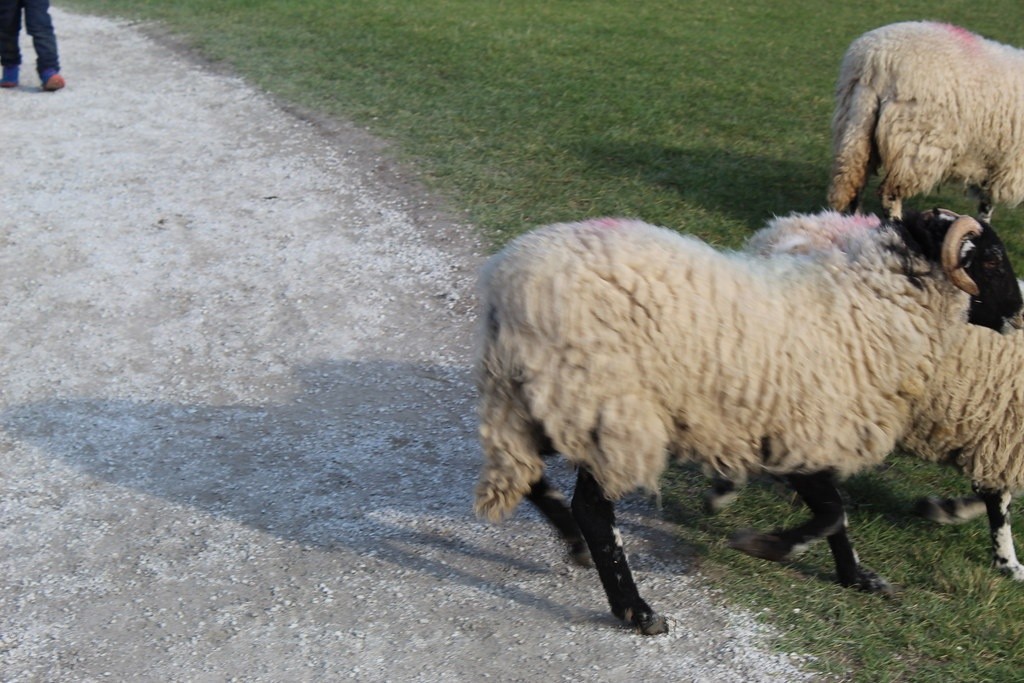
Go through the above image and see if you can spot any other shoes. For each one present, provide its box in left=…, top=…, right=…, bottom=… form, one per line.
left=0, top=64, right=20, bottom=86
left=41, top=67, right=65, bottom=90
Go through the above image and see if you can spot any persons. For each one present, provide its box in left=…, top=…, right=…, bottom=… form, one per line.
left=0, top=0, right=65, bottom=91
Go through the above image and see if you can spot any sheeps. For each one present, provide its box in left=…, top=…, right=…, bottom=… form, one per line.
left=473, top=206, right=1024, bottom=637
left=821, top=13, right=1024, bottom=222
